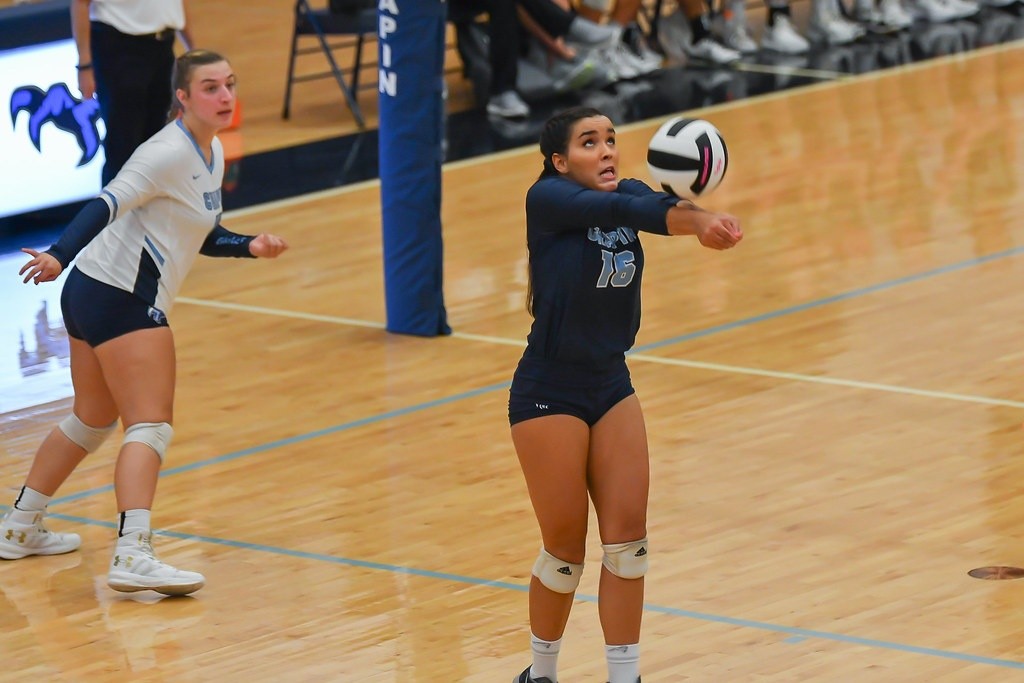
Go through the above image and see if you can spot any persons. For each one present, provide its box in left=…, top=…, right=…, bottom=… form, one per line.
left=507, top=109, right=743, bottom=683
left=68, top=0, right=198, bottom=192
left=444, top=0, right=1024, bottom=118
left=1, top=50, right=290, bottom=597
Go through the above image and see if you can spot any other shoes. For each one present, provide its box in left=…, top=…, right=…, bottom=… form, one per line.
left=810, top=0, right=980, bottom=46
left=543, top=41, right=662, bottom=92
left=487, top=89, right=530, bottom=118
left=717, top=25, right=758, bottom=54
left=682, top=34, right=740, bottom=64
left=567, top=17, right=621, bottom=49
left=760, top=13, right=809, bottom=54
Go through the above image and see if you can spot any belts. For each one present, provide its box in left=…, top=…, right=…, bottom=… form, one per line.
left=91, top=22, right=174, bottom=42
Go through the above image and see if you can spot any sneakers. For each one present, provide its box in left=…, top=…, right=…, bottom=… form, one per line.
left=511, top=663, right=560, bottom=683
left=107, top=530, right=205, bottom=595
left=0, top=506, right=80, bottom=560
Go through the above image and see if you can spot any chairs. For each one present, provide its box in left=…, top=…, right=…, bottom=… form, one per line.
left=281, top=0, right=378, bottom=131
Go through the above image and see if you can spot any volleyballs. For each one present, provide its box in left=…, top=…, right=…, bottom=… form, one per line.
left=648, top=114, right=731, bottom=197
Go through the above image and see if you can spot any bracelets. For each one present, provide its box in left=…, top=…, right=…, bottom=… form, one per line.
left=75, top=61, right=92, bottom=70
